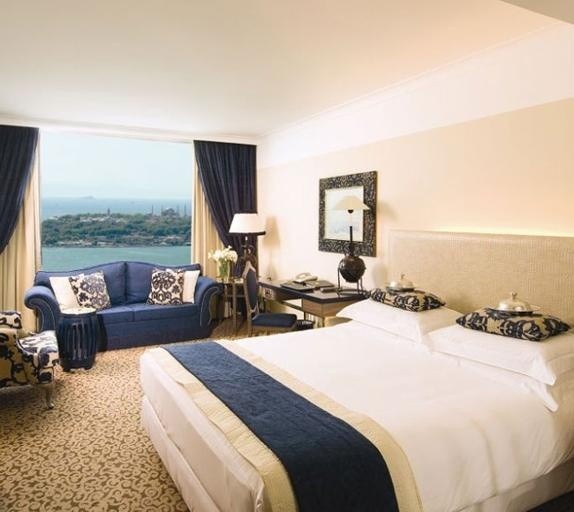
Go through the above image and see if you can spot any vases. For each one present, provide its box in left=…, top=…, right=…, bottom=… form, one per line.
left=216, top=262, right=229, bottom=284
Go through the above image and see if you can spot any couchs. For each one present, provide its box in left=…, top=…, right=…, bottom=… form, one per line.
left=24, top=261, right=224, bottom=350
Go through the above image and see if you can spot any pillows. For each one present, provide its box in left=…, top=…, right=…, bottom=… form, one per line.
left=35, top=260, right=201, bottom=311
left=457, top=306, right=571, bottom=340
left=369, top=288, right=448, bottom=312
left=422, top=320, right=574, bottom=385
left=335, top=297, right=465, bottom=340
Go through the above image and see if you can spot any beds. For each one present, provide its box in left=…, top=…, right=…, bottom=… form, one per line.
left=138, top=233, right=574, bottom=512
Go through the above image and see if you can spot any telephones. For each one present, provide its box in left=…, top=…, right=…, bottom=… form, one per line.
left=294, top=272, right=318, bottom=284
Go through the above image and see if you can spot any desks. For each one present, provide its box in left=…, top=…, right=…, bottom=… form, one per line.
left=257, top=280, right=367, bottom=327
left=214, top=277, right=270, bottom=336
left=56, top=307, right=99, bottom=371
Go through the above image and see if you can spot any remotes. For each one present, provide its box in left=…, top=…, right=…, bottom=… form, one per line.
left=320, top=285, right=342, bottom=293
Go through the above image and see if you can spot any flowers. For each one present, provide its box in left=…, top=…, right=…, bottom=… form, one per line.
left=207, top=245, right=238, bottom=281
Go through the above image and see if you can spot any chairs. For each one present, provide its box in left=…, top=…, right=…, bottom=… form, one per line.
left=242, top=261, right=298, bottom=335
left=0, top=310, right=59, bottom=409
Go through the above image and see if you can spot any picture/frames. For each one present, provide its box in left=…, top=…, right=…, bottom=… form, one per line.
left=318, top=170, right=377, bottom=257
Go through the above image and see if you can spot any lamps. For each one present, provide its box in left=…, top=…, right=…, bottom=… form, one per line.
left=227, top=213, right=266, bottom=284
left=331, top=195, right=371, bottom=297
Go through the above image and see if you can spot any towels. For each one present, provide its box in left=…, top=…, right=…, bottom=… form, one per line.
left=153, top=337, right=423, bottom=512
